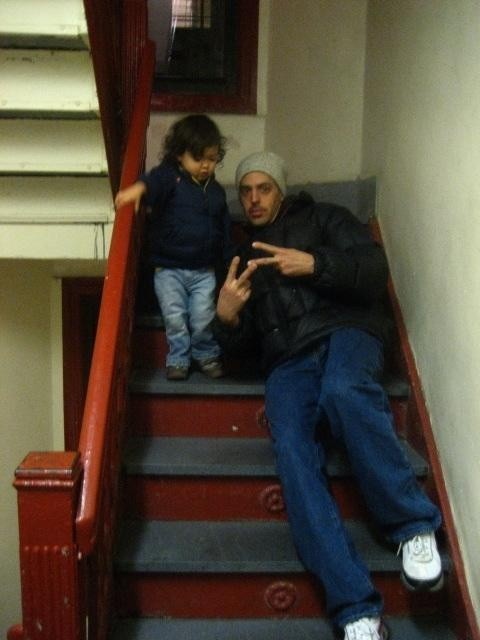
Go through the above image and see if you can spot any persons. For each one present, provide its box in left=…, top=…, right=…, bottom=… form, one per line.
left=216, top=152, right=444, bottom=640
left=115, top=115, right=226, bottom=377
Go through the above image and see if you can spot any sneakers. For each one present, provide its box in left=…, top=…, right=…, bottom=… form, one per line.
left=343, top=616, right=389, bottom=640
left=200, top=358, right=225, bottom=377
left=402, top=530, right=445, bottom=592
left=166, top=366, right=188, bottom=379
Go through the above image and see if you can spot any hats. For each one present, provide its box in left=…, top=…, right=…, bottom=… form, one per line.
left=235, top=152, right=289, bottom=196
